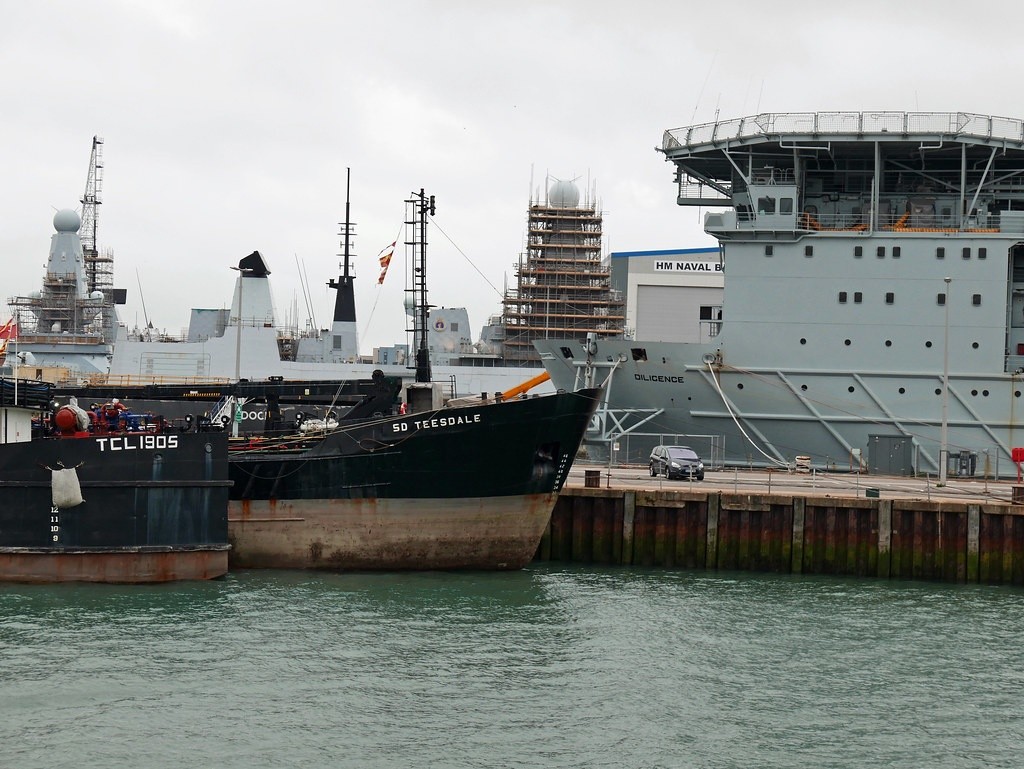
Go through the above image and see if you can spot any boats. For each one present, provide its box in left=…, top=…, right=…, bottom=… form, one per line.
left=0, top=133, right=607, bottom=584
left=530, top=106, right=1024, bottom=481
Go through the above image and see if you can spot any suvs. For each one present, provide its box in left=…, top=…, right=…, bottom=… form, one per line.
left=648, top=444, right=705, bottom=481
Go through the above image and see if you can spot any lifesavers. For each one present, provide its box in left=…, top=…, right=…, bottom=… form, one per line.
left=400, top=402, right=407, bottom=414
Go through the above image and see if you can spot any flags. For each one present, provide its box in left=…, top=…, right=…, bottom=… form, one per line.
left=1, top=310, right=18, bottom=405
left=377, top=240, right=396, bottom=282
